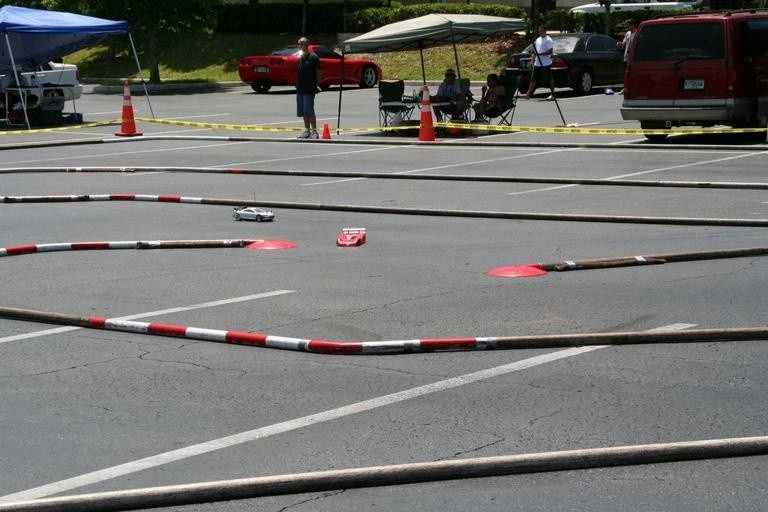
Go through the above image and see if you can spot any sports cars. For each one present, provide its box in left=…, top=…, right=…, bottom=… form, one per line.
left=238, top=44, right=382, bottom=94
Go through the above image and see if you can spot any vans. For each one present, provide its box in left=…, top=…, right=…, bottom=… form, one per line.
left=620, top=9, right=768, bottom=143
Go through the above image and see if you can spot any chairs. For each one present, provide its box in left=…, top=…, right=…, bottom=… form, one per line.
left=372, top=70, right=522, bottom=139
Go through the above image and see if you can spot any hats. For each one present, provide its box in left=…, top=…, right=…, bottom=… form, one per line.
left=445, top=68, right=455, bottom=75
left=11, top=64, right=24, bottom=72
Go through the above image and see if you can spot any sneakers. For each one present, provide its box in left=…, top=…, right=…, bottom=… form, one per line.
left=546, top=94, right=554, bottom=100
left=298, top=131, right=319, bottom=139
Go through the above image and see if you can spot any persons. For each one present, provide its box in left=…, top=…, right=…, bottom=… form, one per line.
left=524, top=25, right=556, bottom=101
left=0, top=63, right=39, bottom=119
left=295, top=37, right=320, bottom=140
left=616, top=19, right=638, bottom=94
left=429, top=68, right=467, bottom=123
left=472, top=74, right=507, bottom=125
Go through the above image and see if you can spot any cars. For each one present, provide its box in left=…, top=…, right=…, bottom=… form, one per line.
left=0, top=56, right=83, bottom=116
left=569, top=0, right=704, bottom=15
left=505, top=33, right=625, bottom=95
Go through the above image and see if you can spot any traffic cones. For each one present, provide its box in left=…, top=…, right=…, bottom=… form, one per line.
left=413, top=85, right=442, bottom=144
left=321, top=123, right=333, bottom=139
left=115, top=80, right=143, bottom=136
